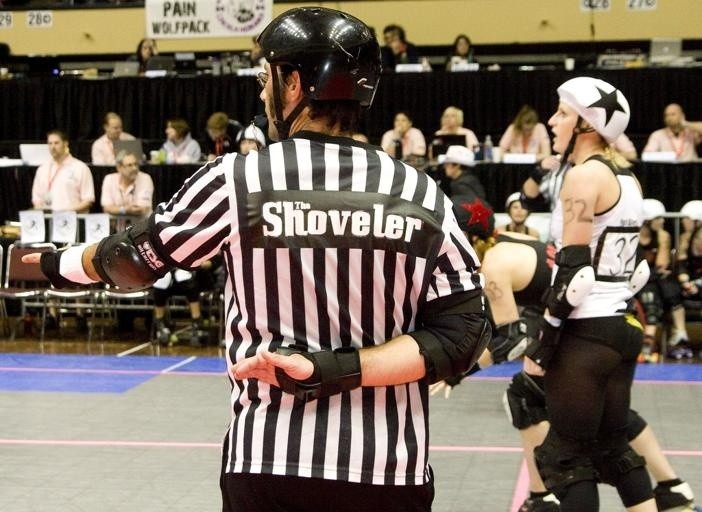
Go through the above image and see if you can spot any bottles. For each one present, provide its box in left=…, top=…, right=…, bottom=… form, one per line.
left=158, top=147, right=167, bottom=164
left=483, top=135, right=493, bottom=163
left=394, top=139, right=402, bottom=161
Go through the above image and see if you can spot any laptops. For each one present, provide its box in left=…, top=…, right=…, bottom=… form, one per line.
left=112, top=61, right=140, bottom=78
left=649, top=37, right=683, bottom=64
left=19, top=143, right=53, bottom=166
left=112, top=138, right=147, bottom=162
left=432, top=133, right=469, bottom=161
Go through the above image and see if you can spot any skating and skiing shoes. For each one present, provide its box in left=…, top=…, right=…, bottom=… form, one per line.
left=155, top=317, right=178, bottom=347
left=638, top=343, right=662, bottom=363
left=668, top=334, right=694, bottom=360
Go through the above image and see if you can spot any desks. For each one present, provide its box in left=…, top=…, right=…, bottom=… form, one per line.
left=1, top=40, right=270, bottom=210
left=359, top=38, right=702, bottom=246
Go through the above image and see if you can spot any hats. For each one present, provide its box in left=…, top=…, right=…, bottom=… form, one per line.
left=438, top=147, right=476, bottom=167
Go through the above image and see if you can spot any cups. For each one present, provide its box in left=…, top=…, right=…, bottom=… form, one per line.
left=150, top=150, right=158, bottom=163
left=565, top=57, right=575, bottom=71
left=491, top=147, right=501, bottom=163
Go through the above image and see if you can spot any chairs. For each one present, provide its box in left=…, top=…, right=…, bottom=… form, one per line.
left=655, top=250, right=702, bottom=346
left=0, top=210, right=226, bottom=347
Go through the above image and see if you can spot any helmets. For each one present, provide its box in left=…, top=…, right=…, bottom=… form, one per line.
left=642, top=198, right=667, bottom=224
left=557, top=76, right=631, bottom=145
left=679, top=199, right=702, bottom=221
left=236, top=126, right=266, bottom=149
left=256, top=8, right=382, bottom=111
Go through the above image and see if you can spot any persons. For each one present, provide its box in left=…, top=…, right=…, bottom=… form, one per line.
left=450, top=194, right=702, bottom=511
left=21, top=6, right=498, bottom=512
left=523, top=76, right=658, bottom=511
